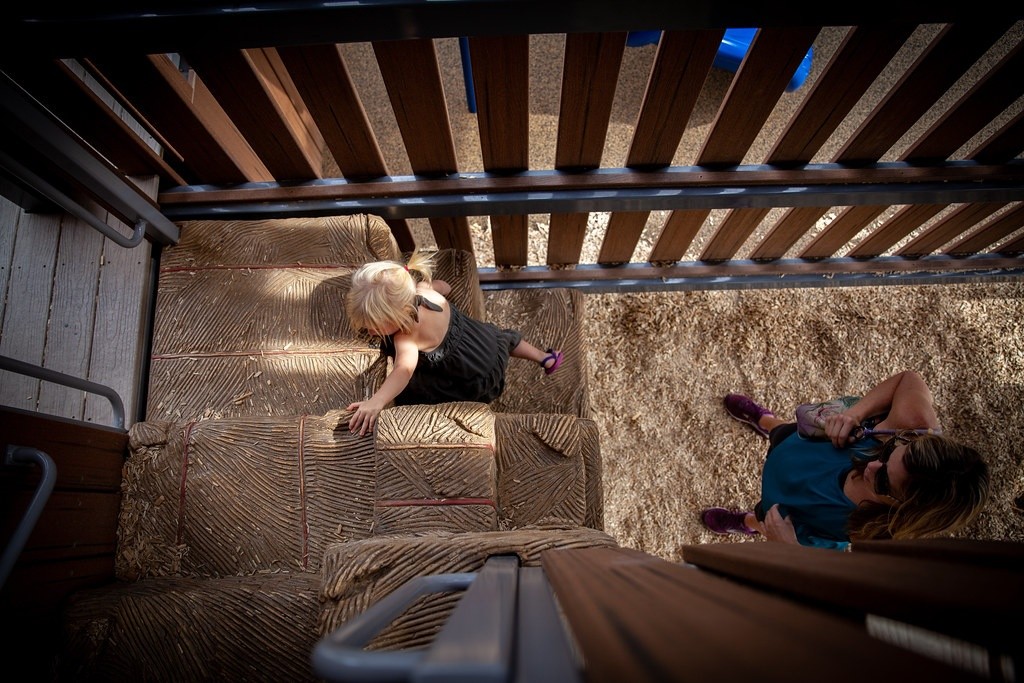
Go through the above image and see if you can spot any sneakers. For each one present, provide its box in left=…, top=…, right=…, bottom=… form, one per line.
left=724, top=393, right=775, bottom=439
left=701, top=506, right=761, bottom=538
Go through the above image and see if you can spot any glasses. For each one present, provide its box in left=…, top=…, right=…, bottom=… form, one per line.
left=873, top=435, right=911, bottom=504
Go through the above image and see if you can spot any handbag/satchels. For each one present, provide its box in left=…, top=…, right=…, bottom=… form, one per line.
left=796, top=395, right=943, bottom=445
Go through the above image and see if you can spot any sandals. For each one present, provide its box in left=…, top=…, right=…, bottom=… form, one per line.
left=540, top=349, right=564, bottom=375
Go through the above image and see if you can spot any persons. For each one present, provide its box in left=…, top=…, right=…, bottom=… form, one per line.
left=699, top=372, right=990, bottom=550
left=346, top=259, right=565, bottom=436
